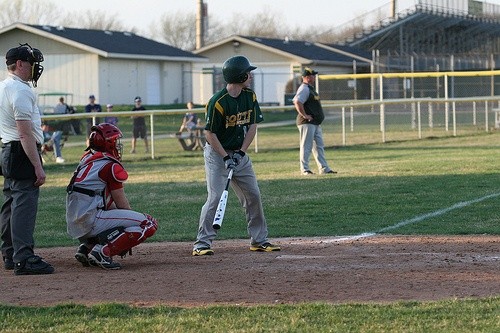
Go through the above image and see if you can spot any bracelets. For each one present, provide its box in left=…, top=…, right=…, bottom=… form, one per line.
left=235, top=149, right=245, bottom=157
left=223, top=155, right=231, bottom=162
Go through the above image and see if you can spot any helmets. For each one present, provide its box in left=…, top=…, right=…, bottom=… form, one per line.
left=222, top=57, right=258, bottom=84
left=89, top=123, right=123, bottom=151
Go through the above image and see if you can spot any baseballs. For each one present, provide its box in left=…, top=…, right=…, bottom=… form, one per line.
left=212, top=170, right=234, bottom=229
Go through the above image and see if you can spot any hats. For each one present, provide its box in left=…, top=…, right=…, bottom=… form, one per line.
left=106, top=104, right=113, bottom=108
left=6, top=48, right=35, bottom=65
left=88, top=95, right=95, bottom=100
left=302, top=68, right=319, bottom=77
left=134, top=96, right=142, bottom=102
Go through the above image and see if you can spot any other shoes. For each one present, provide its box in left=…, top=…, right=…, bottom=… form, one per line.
left=130, top=147, right=149, bottom=154
left=327, top=170, right=337, bottom=174
left=302, top=171, right=316, bottom=175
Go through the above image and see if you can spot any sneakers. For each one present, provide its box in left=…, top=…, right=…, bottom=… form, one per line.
left=192, top=248, right=215, bottom=256
left=14, top=254, right=54, bottom=275
left=56, top=156, right=66, bottom=163
left=75, top=243, right=92, bottom=266
left=88, top=244, right=121, bottom=270
left=4, top=258, right=15, bottom=270
left=249, top=242, right=281, bottom=252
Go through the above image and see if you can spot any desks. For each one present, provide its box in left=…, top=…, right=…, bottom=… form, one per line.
left=175, top=124, right=208, bottom=152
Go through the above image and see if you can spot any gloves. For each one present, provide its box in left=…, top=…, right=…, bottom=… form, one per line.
left=223, top=150, right=246, bottom=169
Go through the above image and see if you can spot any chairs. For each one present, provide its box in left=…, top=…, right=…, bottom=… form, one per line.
left=40, top=135, right=69, bottom=164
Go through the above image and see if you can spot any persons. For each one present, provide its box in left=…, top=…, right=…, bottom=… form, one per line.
left=192, top=56, right=281, bottom=256
left=103, top=104, right=118, bottom=127
left=85, top=95, right=102, bottom=148
left=39, top=97, right=83, bottom=164
left=292, top=68, right=338, bottom=174
left=178, top=102, right=198, bottom=143
left=129, top=97, right=150, bottom=153
left=0, top=42, right=54, bottom=275
left=65, top=123, right=158, bottom=271
